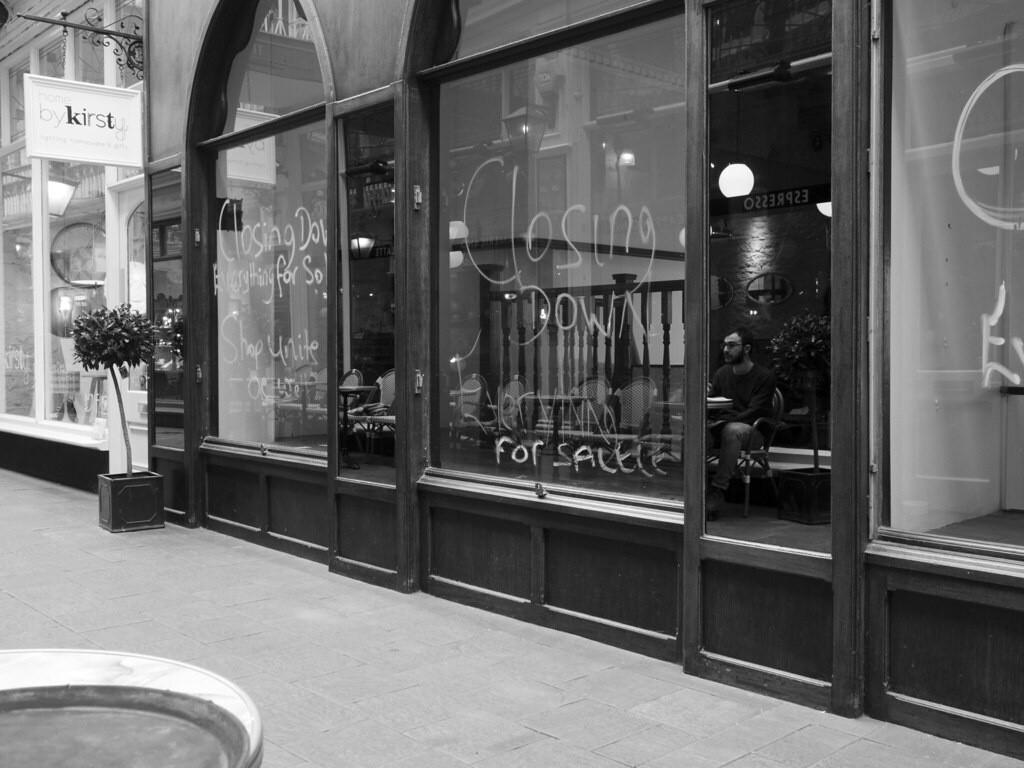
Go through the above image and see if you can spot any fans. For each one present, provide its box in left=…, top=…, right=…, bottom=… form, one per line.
left=50, top=223, right=107, bottom=290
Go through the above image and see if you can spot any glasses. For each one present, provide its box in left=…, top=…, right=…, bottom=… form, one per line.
left=719, top=341, right=744, bottom=349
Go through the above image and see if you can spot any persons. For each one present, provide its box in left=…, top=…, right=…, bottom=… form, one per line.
left=700, top=327, right=775, bottom=513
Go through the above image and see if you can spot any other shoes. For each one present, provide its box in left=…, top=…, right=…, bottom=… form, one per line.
left=706, top=491, right=721, bottom=522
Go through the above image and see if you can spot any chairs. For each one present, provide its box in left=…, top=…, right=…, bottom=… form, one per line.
left=566, top=375, right=664, bottom=477
left=258, top=360, right=396, bottom=473
left=453, top=374, right=528, bottom=465
left=707, top=389, right=782, bottom=518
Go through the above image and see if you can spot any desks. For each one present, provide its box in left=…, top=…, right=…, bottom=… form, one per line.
left=451, top=390, right=479, bottom=447
left=607, top=395, right=735, bottom=487
left=282, top=381, right=327, bottom=442
left=339, top=385, right=375, bottom=470
left=523, top=395, right=594, bottom=466
left=81, top=374, right=109, bottom=425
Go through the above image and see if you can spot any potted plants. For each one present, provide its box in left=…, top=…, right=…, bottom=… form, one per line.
left=772, top=313, right=831, bottom=526
left=72, top=303, right=167, bottom=533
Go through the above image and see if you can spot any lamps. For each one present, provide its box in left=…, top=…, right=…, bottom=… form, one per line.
left=775, top=64, right=794, bottom=80
left=718, top=94, right=755, bottom=199
